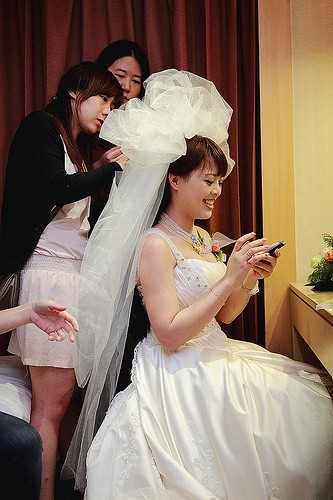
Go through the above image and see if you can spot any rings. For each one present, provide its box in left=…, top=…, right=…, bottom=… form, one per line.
left=245, top=250, right=252, bottom=258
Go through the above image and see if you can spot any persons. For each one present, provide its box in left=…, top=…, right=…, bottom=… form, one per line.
left=0, top=300, right=79, bottom=500
left=91, top=39, right=150, bottom=169
left=0, top=61, right=132, bottom=500
left=84, top=135, right=333, bottom=500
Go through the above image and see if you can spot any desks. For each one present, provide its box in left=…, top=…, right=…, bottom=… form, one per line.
left=288, top=282, right=333, bottom=379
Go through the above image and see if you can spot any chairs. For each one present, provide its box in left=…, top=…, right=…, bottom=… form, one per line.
left=0, top=411, right=45, bottom=500
left=0, top=354, right=34, bottom=423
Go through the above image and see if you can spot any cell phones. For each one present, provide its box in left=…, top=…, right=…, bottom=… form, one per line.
left=258, top=243, right=286, bottom=264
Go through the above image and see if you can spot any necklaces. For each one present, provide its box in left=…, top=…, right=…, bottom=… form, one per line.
left=158, top=212, right=207, bottom=258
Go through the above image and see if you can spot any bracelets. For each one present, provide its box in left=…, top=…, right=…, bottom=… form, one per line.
left=240, top=283, right=252, bottom=292
left=210, top=288, right=225, bottom=305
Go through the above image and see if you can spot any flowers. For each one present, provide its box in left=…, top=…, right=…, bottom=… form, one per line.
left=197, top=227, right=224, bottom=263
left=303, top=232, right=333, bottom=293
